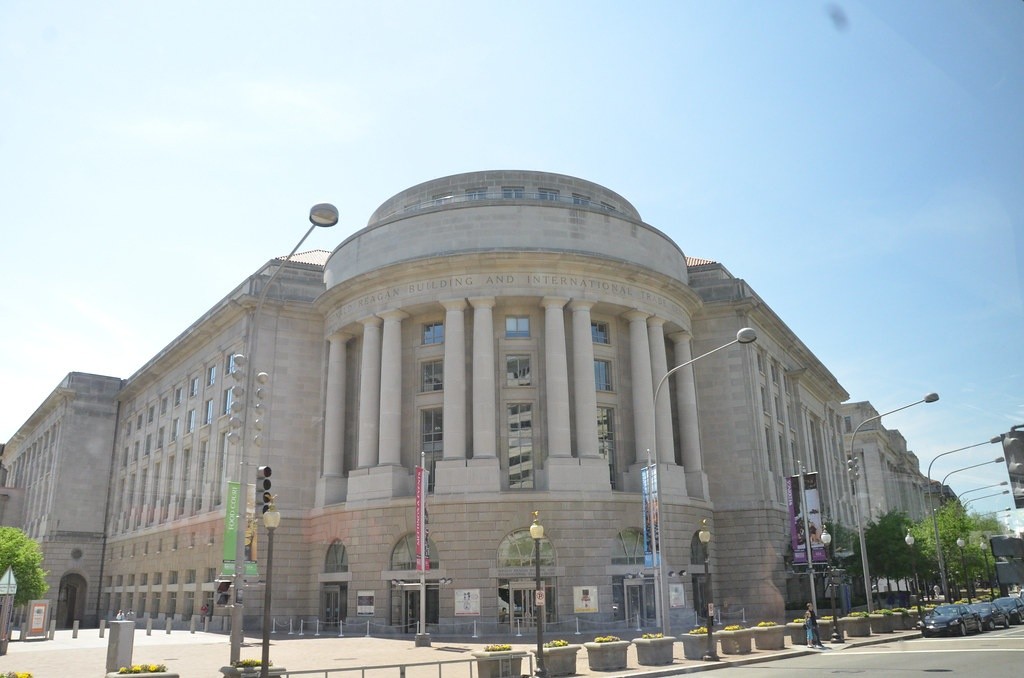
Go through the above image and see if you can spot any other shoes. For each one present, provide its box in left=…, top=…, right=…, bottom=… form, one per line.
left=816, top=644, right=822, bottom=648
left=808, top=644, right=813, bottom=647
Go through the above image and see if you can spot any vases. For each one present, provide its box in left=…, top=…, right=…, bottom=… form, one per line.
left=104, top=670, right=179, bottom=678
left=632, top=635, right=677, bottom=665
left=471, top=650, right=527, bottom=678
left=584, top=640, right=630, bottom=671
left=753, top=624, right=787, bottom=650
left=787, top=610, right=934, bottom=644
left=530, top=644, right=580, bottom=677
left=221, top=666, right=286, bottom=678
left=682, top=631, right=721, bottom=659
left=717, top=627, right=758, bottom=654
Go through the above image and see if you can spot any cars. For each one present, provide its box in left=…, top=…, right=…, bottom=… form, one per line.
left=992, top=589, right=1024, bottom=625
left=919, top=603, right=984, bottom=637
left=967, top=601, right=1011, bottom=631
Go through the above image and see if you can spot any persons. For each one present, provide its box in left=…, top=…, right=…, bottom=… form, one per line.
left=805, top=603, right=822, bottom=648
left=809, top=522, right=816, bottom=542
left=201, top=603, right=212, bottom=629
left=116, top=610, right=125, bottom=621
left=126, top=609, right=136, bottom=620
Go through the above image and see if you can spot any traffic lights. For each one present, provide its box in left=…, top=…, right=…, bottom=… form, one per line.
left=214, top=579, right=233, bottom=605
left=254, top=464, right=272, bottom=518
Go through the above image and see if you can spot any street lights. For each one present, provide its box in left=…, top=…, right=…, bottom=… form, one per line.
left=850, top=394, right=941, bottom=614
left=904, top=531, right=923, bottom=620
left=820, top=525, right=844, bottom=642
left=260, top=494, right=282, bottom=678
left=529, top=510, right=552, bottom=678
left=956, top=537, right=972, bottom=604
left=228, top=203, right=342, bottom=669
left=651, top=327, right=758, bottom=641
left=980, top=542, right=996, bottom=598
left=939, top=456, right=1006, bottom=508
left=697, top=518, right=720, bottom=662
left=928, top=436, right=1003, bottom=603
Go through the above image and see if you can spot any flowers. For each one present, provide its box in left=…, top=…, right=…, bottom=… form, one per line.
left=724, top=623, right=744, bottom=631
left=793, top=594, right=994, bottom=623
left=484, top=643, right=512, bottom=652
left=757, top=621, right=779, bottom=627
left=689, top=626, right=712, bottom=634
left=0, top=671, right=34, bottom=678
left=595, top=635, right=621, bottom=643
left=642, top=632, right=665, bottom=639
left=118, top=661, right=167, bottom=675
left=543, top=638, right=568, bottom=647
left=231, top=659, right=271, bottom=667
